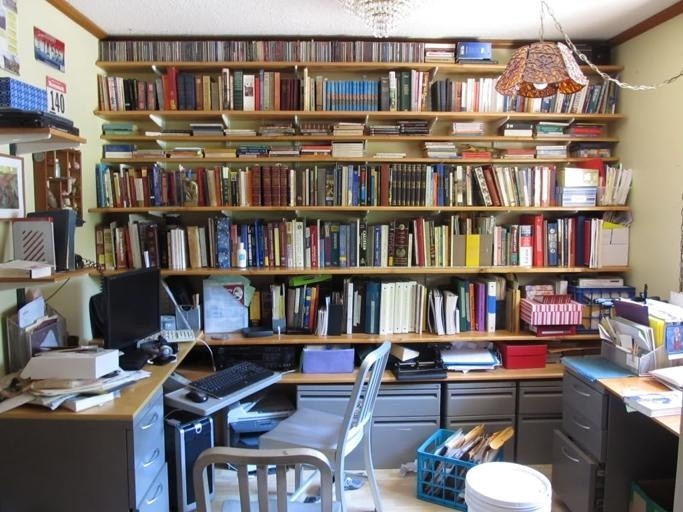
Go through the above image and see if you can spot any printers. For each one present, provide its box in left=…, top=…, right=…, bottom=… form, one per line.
left=224, top=393, right=296, bottom=474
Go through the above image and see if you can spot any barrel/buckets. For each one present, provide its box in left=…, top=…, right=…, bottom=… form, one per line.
left=464, top=461, right=554, bottom=512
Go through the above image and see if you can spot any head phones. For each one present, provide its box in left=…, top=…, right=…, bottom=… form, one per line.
left=140, top=340, right=178, bottom=358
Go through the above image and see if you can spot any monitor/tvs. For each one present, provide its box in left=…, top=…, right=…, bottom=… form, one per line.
left=103, top=267, right=161, bottom=370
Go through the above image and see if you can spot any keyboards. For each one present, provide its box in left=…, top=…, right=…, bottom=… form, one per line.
left=187, top=360, right=274, bottom=400
left=137, top=329, right=195, bottom=348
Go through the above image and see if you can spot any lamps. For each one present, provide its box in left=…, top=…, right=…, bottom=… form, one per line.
left=494, top=3, right=588, bottom=100
left=335, top=0, right=415, bottom=38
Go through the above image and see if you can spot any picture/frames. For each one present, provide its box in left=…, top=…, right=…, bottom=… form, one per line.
left=0, top=153, right=27, bottom=222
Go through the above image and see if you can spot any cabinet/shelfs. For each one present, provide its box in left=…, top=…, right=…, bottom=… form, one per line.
left=32, top=148, right=83, bottom=227
left=1, top=126, right=97, bottom=293
left=88, top=38, right=636, bottom=385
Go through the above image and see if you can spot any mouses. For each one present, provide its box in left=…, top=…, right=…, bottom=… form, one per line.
left=187, top=390, right=208, bottom=403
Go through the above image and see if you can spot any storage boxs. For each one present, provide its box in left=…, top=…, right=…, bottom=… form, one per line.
left=627, top=477, right=676, bottom=512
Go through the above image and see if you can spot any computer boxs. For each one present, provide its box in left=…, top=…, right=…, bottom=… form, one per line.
left=165, top=410, right=215, bottom=512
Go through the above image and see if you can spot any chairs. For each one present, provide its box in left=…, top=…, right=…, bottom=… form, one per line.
left=193, top=445, right=334, bottom=512
left=258, top=339, right=391, bottom=511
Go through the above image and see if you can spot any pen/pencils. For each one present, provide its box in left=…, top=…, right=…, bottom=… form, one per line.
left=192, top=292, right=199, bottom=308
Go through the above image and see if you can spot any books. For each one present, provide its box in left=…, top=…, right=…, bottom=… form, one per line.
left=95, top=160, right=633, bottom=208
left=98, top=40, right=493, bottom=63
left=0, top=346, right=151, bottom=413
left=98, top=69, right=618, bottom=114
left=96, top=214, right=629, bottom=270
left=597, top=291, right=683, bottom=417
left=103, top=122, right=612, bottom=159
left=204, top=274, right=630, bottom=335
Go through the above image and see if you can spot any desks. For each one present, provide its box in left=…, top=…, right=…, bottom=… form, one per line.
left=1, top=328, right=204, bottom=512
left=551, top=355, right=682, bottom=512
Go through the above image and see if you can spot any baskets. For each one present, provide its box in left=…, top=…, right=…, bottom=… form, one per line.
left=417, top=430, right=504, bottom=511
